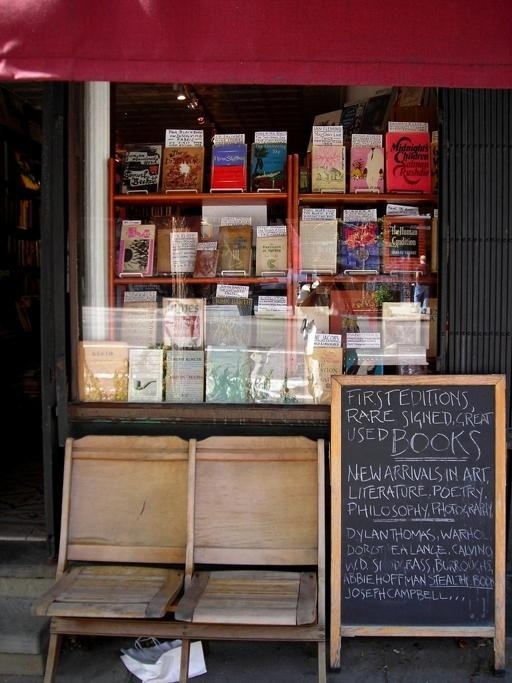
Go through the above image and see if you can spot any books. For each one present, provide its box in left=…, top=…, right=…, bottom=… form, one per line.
left=75, top=87, right=439, bottom=408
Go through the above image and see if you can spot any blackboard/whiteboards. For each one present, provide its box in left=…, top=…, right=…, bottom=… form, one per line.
left=340, top=386, right=496, bottom=628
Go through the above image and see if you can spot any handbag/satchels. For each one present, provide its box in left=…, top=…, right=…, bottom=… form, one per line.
left=120, top=639, right=208, bottom=683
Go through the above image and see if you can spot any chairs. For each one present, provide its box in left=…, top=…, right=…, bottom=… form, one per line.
left=30, top=434, right=327, bottom=682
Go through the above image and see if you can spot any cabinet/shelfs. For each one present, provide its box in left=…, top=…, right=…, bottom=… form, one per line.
left=107, top=154, right=439, bottom=377
